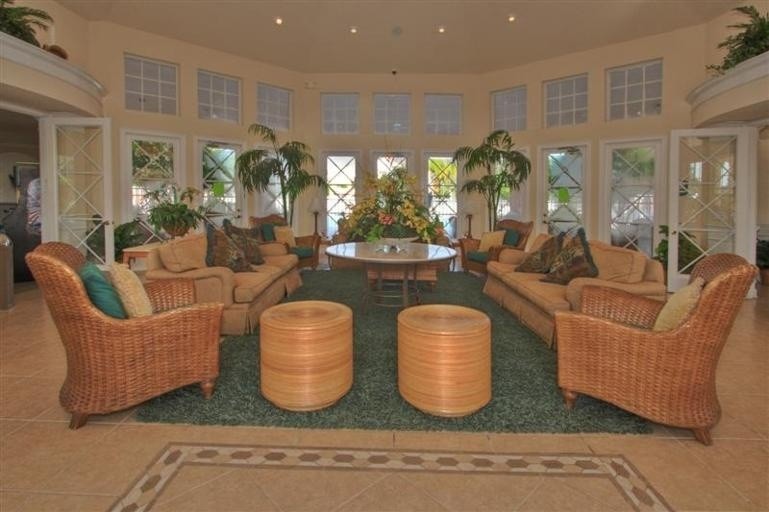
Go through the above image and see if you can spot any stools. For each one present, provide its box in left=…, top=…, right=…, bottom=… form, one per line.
left=397, top=304, right=493, bottom=418
left=258, top=301, right=354, bottom=412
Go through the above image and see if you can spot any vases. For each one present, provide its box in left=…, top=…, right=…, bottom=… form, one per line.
left=381, top=236, right=420, bottom=243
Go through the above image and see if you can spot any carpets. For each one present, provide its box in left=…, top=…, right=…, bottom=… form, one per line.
left=134, top=270, right=656, bottom=436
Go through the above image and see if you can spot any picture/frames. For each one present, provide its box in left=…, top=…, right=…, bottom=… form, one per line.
left=15, top=161, right=40, bottom=189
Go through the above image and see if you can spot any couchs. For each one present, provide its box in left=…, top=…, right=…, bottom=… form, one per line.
left=124, top=229, right=302, bottom=336
left=483, top=232, right=668, bottom=353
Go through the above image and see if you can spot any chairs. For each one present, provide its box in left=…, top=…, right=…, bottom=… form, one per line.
left=554, top=253, right=760, bottom=445
left=249, top=215, right=321, bottom=270
left=25, top=242, right=225, bottom=430
left=458, top=218, right=534, bottom=276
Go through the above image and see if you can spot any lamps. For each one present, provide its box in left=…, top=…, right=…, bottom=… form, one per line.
left=308, top=198, right=322, bottom=235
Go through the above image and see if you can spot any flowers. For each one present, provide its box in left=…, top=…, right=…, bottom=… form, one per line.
left=335, top=165, right=446, bottom=245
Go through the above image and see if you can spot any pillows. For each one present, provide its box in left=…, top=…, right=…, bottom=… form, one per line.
left=206, top=219, right=298, bottom=272
left=78, top=258, right=126, bottom=319
left=653, top=275, right=706, bottom=331
left=478, top=227, right=598, bottom=285
left=109, top=261, right=152, bottom=319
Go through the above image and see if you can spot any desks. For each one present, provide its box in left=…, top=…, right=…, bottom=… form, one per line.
left=122, top=241, right=166, bottom=269
left=367, top=264, right=438, bottom=294
left=325, top=241, right=457, bottom=309
